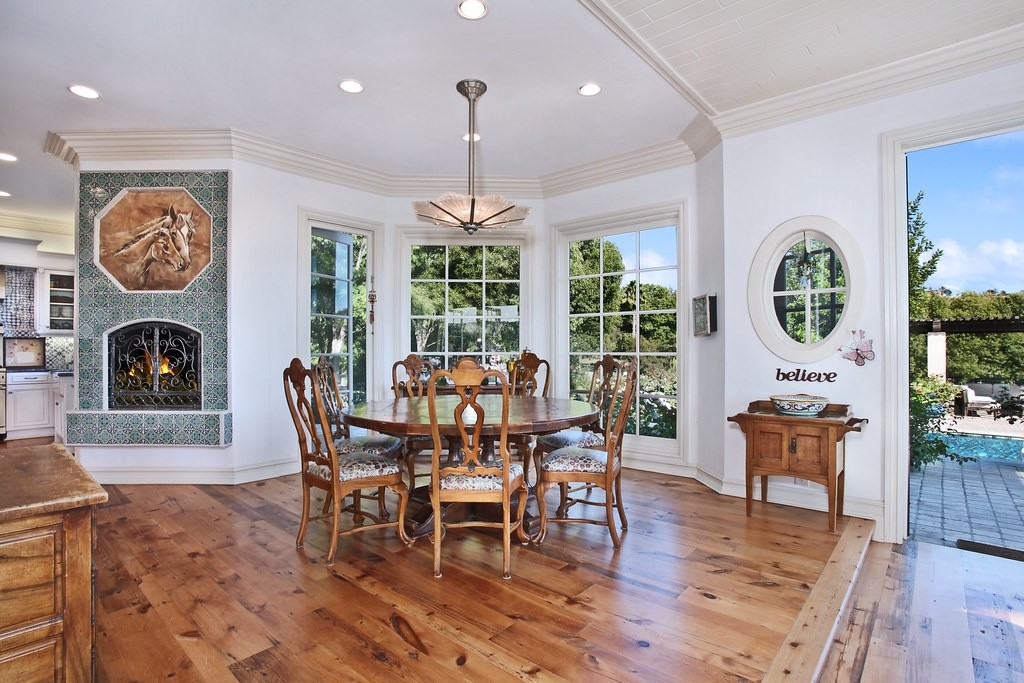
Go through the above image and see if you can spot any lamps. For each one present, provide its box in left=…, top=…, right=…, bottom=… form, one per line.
left=411, top=79, right=531, bottom=235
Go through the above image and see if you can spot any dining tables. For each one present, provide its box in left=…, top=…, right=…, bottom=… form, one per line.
left=390, top=382, right=537, bottom=398
left=339, top=392, right=602, bottom=543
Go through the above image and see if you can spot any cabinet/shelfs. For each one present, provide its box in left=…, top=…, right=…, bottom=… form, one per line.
left=44, top=269, right=74, bottom=334
left=726, top=400, right=868, bottom=533
left=1, top=443, right=109, bottom=683
left=54, top=377, right=74, bottom=443
left=6, top=371, right=55, bottom=440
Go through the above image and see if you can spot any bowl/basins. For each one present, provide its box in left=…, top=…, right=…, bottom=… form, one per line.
left=769, top=394, right=829, bottom=416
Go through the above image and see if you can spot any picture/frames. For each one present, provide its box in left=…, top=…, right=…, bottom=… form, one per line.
left=92, top=186, right=214, bottom=294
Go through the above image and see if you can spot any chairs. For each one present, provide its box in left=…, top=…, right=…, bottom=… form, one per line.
left=312, top=355, right=407, bottom=523
left=426, top=356, right=530, bottom=580
left=283, top=357, right=415, bottom=567
left=532, top=354, right=623, bottom=495
left=531, top=363, right=637, bottom=551
left=495, top=351, right=551, bottom=489
left=392, top=354, right=450, bottom=499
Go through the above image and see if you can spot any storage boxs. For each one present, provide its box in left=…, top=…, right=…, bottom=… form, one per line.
left=768, top=393, right=830, bottom=418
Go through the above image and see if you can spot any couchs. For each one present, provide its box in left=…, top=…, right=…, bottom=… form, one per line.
left=954, top=383, right=1024, bottom=419
